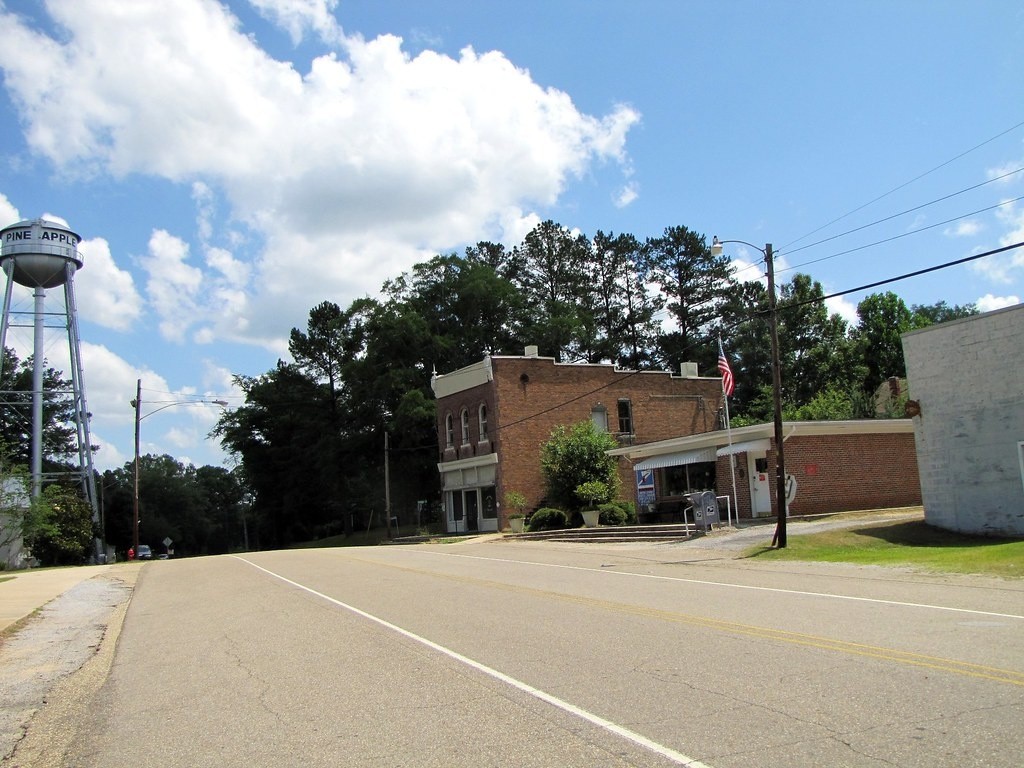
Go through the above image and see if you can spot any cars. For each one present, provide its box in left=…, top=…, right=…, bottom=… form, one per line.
left=136, top=545, right=153, bottom=559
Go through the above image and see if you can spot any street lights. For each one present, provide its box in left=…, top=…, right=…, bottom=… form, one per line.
left=130, top=399, right=228, bottom=560
left=710, top=236, right=788, bottom=547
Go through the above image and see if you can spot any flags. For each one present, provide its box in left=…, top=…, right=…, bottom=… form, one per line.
left=718, top=336, right=735, bottom=397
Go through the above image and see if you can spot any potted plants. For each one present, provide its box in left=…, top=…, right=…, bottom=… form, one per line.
left=648, top=501, right=656, bottom=512
left=573, top=481, right=608, bottom=528
left=504, top=490, right=527, bottom=533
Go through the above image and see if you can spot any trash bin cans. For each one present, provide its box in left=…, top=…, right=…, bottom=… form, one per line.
left=690, top=491, right=722, bottom=535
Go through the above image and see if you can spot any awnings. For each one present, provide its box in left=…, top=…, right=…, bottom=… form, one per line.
left=632, top=446, right=718, bottom=471
left=716, top=438, right=772, bottom=457
